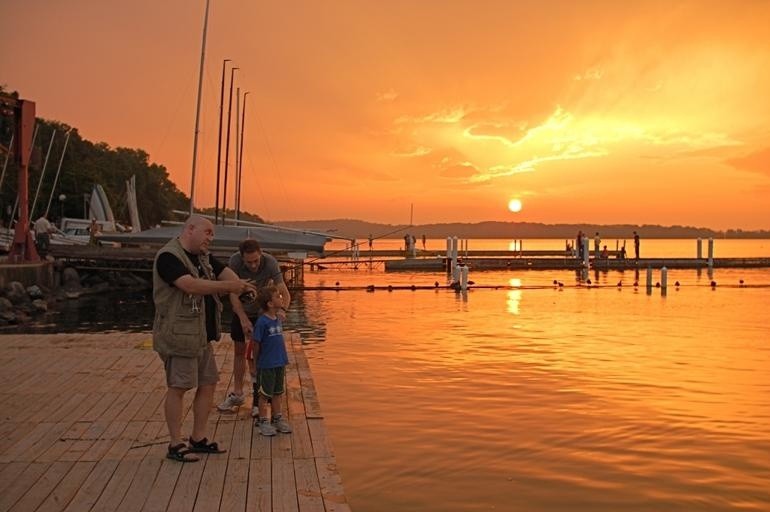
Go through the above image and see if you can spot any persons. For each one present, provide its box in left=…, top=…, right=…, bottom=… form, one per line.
left=617, top=247, right=627, bottom=259
left=403, top=233, right=410, bottom=250
left=33, top=211, right=53, bottom=259
left=150, top=216, right=257, bottom=463
left=632, top=230, right=640, bottom=259
left=368, top=233, right=374, bottom=250
left=576, top=230, right=583, bottom=257
left=601, top=245, right=608, bottom=259
left=594, top=232, right=601, bottom=259
left=248, top=285, right=293, bottom=437
left=581, top=231, right=589, bottom=260
left=86, top=217, right=100, bottom=246
left=214, top=237, right=291, bottom=419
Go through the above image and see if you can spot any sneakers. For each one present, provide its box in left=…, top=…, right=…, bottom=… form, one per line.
left=272, top=417, right=293, bottom=433
left=258, top=421, right=277, bottom=436
left=251, top=406, right=259, bottom=417
left=216, top=393, right=246, bottom=411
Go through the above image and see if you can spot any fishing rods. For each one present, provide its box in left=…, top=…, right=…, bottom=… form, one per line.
left=233, top=222, right=414, bottom=303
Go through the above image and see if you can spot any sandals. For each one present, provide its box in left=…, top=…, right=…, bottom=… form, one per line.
left=188, top=436, right=227, bottom=454
left=166, top=443, right=200, bottom=462
